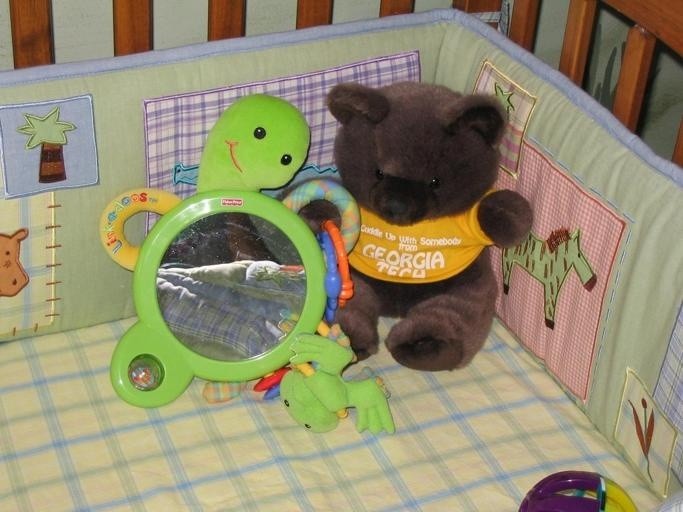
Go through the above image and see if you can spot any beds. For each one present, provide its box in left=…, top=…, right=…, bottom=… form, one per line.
left=0, top=0, right=683, bottom=512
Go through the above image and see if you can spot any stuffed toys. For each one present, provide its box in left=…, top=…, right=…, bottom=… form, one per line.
left=274, top=81, right=531, bottom=370
left=280, top=322, right=395, bottom=435
left=197, top=94, right=310, bottom=193
